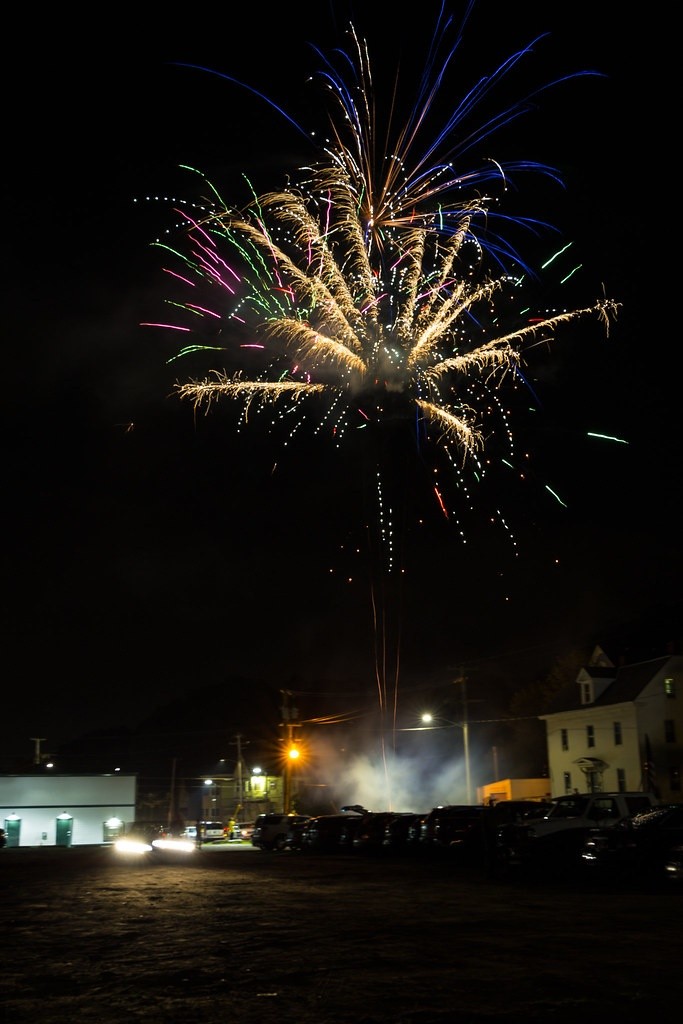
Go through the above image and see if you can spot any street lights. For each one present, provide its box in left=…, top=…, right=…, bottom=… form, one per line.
left=220, top=758, right=242, bottom=806
left=419, top=711, right=474, bottom=806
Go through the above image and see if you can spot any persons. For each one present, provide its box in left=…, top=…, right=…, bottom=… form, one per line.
left=228, top=818, right=236, bottom=840
left=195, top=819, right=203, bottom=849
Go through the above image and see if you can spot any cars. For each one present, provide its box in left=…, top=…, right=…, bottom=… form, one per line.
left=124, top=820, right=173, bottom=865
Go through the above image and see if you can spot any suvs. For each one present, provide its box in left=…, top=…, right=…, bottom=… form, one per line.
left=196, top=820, right=226, bottom=842
left=228, top=791, right=683, bottom=895
